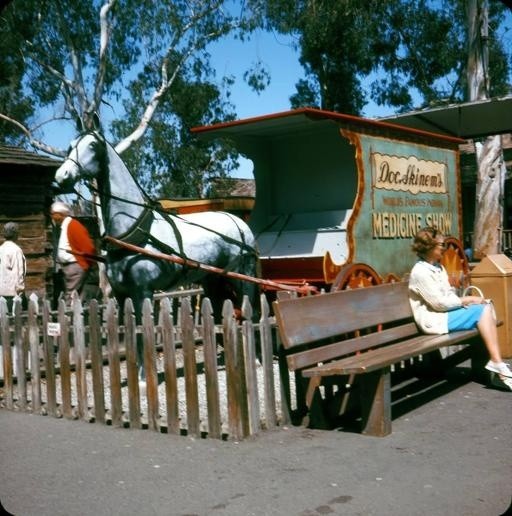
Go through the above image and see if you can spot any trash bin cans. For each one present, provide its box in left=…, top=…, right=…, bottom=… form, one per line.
left=467, top=253, right=512, bottom=358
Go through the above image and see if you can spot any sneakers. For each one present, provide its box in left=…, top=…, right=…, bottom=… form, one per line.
left=485, top=360, right=512, bottom=390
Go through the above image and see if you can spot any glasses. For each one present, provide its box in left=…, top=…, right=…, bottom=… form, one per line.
left=439, top=242, right=445, bottom=247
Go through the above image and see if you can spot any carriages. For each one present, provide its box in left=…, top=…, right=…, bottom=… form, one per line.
left=52, top=106, right=468, bottom=396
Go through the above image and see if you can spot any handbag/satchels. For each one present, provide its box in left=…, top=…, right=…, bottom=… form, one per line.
left=463, top=286, right=496, bottom=321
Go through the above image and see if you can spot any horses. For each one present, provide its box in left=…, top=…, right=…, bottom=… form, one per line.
left=54, top=110, right=263, bottom=387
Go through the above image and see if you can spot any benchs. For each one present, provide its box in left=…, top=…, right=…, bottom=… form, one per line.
left=272, top=279, right=504, bottom=437
left=247, top=208, right=355, bottom=284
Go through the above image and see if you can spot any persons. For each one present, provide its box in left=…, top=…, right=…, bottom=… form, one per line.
left=408, top=227, right=511, bottom=391
left=1, top=222, right=28, bottom=342
left=40, top=201, right=95, bottom=365
